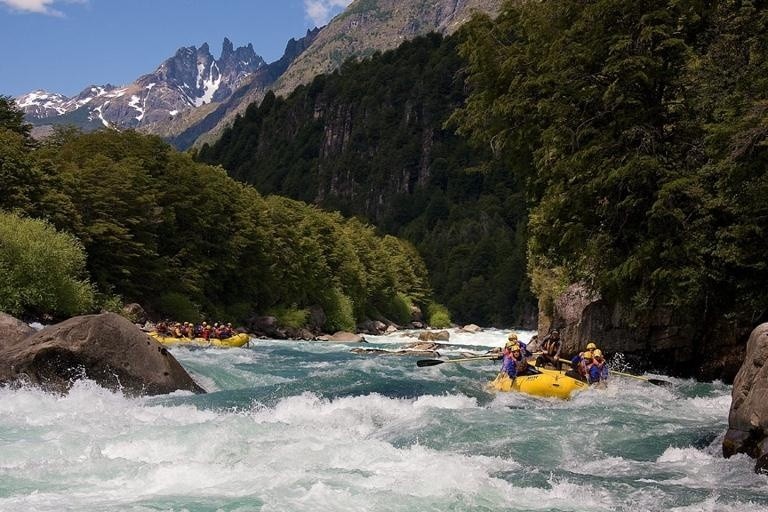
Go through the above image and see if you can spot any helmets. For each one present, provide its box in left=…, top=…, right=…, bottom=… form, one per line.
left=175, top=321, right=232, bottom=330
left=511, top=345, right=520, bottom=352
left=508, top=333, right=519, bottom=341
left=586, top=342, right=597, bottom=349
left=505, top=341, right=514, bottom=347
left=583, top=351, right=592, bottom=359
left=594, top=349, right=602, bottom=357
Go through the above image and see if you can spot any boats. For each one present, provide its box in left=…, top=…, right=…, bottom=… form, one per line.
left=146, top=332, right=250, bottom=348
left=490, top=358, right=590, bottom=403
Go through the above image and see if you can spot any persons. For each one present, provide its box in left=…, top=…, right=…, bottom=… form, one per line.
left=534, top=329, right=563, bottom=370
left=502, top=333, right=543, bottom=380
left=133, top=317, right=234, bottom=342
left=564, top=342, right=609, bottom=388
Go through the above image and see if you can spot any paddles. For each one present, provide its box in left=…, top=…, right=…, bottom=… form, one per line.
left=559, top=359, right=674, bottom=388
left=417, top=356, right=499, bottom=367
